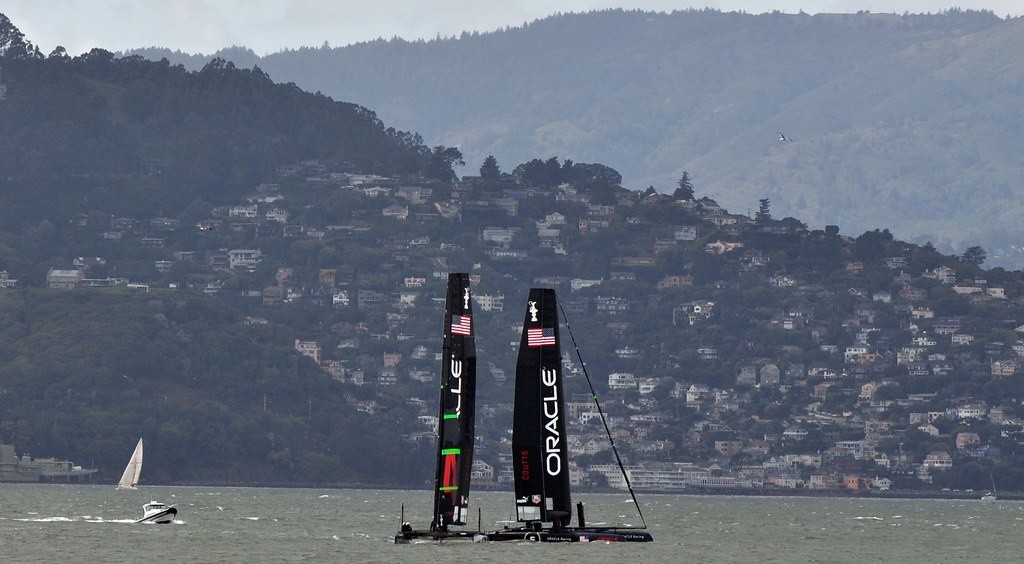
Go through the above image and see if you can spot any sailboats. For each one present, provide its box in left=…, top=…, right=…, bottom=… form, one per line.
left=115, top=436, right=144, bottom=489
left=393, top=271, right=655, bottom=544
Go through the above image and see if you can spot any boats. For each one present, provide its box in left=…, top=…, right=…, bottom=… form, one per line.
left=981, top=493, right=996, bottom=501
left=135, top=501, right=178, bottom=524
